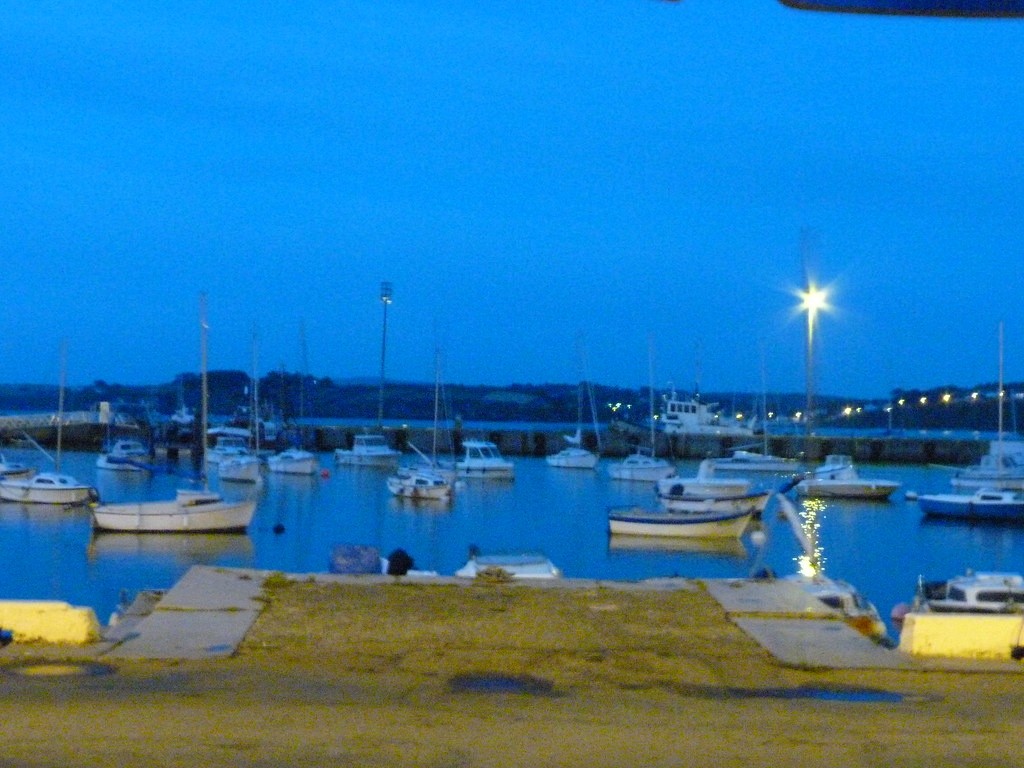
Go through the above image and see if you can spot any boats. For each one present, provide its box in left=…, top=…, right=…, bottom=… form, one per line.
left=458, top=554, right=562, bottom=581
left=795, top=454, right=900, bottom=499
left=96, top=436, right=145, bottom=472
left=383, top=463, right=453, bottom=501
left=656, top=490, right=772, bottom=515
left=705, top=447, right=803, bottom=473
left=608, top=451, right=677, bottom=482
left=915, top=569, right=1024, bottom=613
left=454, top=437, right=515, bottom=481
left=916, top=488, right=1024, bottom=521
left=335, top=431, right=402, bottom=468
left=267, top=442, right=315, bottom=476
left=649, top=459, right=750, bottom=497
left=222, top=451, right=261, bottom=482
left=0, top=453, right=35, bottom=481
left=785, top=576, right=888, bottom=642
left=604, top=504, right=758, bottom=539
left=201, top=421, right=256, bottom=467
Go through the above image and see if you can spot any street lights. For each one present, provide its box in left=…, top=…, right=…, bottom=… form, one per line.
left=801, top=287, right=831, bottom=434
left=377, top=282, right=393, bottom=430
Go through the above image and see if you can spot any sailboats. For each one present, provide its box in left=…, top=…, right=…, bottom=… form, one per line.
left=0, top=337, right=99, bottom=505
left=85, top=290, right=258, bottom=537
left=545, top=336, right=600, bottom=469
left=951, top=315, right=1023, bottom=492
